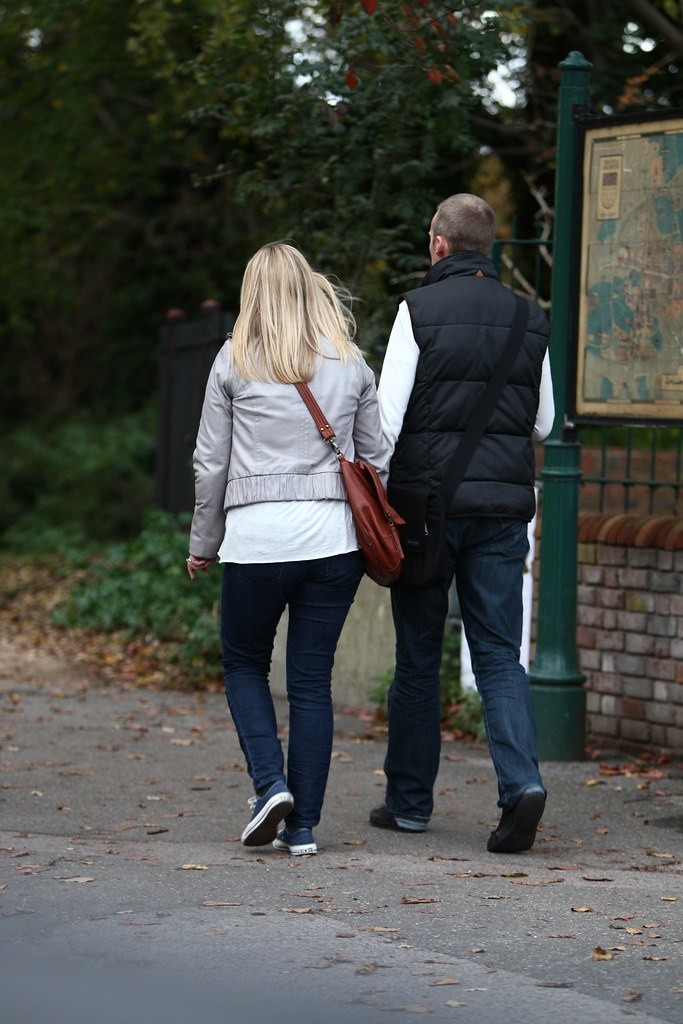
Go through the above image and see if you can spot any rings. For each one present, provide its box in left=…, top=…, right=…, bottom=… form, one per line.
left=186, top=558, right=191, bottom=563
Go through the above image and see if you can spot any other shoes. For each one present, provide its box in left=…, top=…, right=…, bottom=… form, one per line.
left=370, top=804, right=430, bottom=833
left=488, top=787, right=546, bottom=854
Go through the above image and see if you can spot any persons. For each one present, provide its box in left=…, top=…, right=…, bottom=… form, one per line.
left=188, top=239, right=387, bottom=858
left=366, top=192, right=557, bottom=854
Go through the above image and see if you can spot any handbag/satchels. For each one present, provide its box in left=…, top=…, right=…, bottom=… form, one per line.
left=387, top=482, right=447, bottom=591
left=340, top=458, right=405, bottom=587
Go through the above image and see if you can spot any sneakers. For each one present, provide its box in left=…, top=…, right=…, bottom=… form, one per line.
left=241, top=780, right=294, bottom=846
left=272, top=828, right=317, bottom=856
